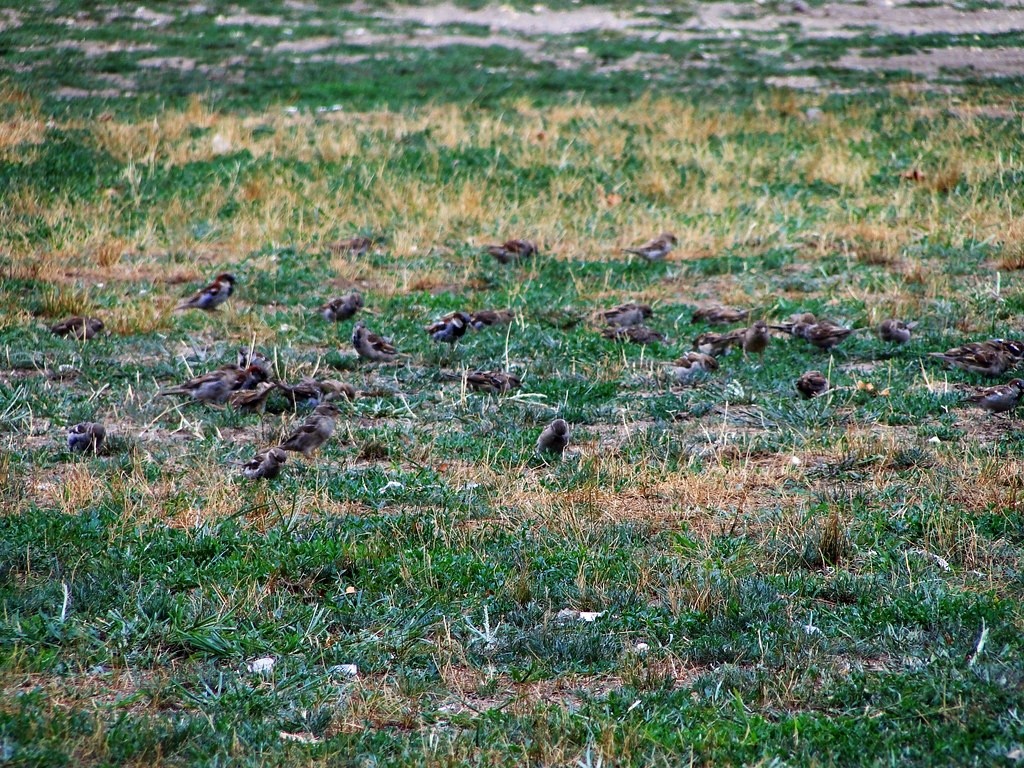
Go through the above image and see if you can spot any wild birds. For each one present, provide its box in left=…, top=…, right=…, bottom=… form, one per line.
left=160, top=231, right=1024, bottom=483
left=50, top=314, right=109, bottom=341
left=67, top=421, right=105, bottom=457
